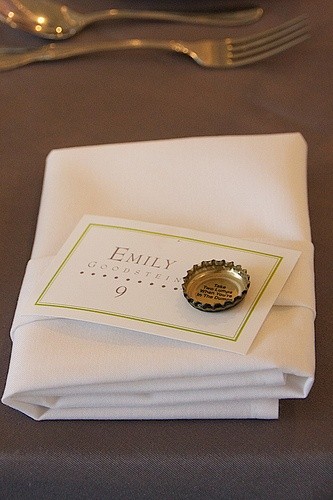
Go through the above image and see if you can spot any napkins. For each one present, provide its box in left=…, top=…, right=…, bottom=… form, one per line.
left=2, top=131, right=315, bottom=421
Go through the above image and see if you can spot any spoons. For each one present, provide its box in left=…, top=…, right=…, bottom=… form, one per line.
left=0, top=0, right=265, bottom=41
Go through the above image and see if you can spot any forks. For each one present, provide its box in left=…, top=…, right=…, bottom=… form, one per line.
left=0, top=12, right=310, bottom=77
